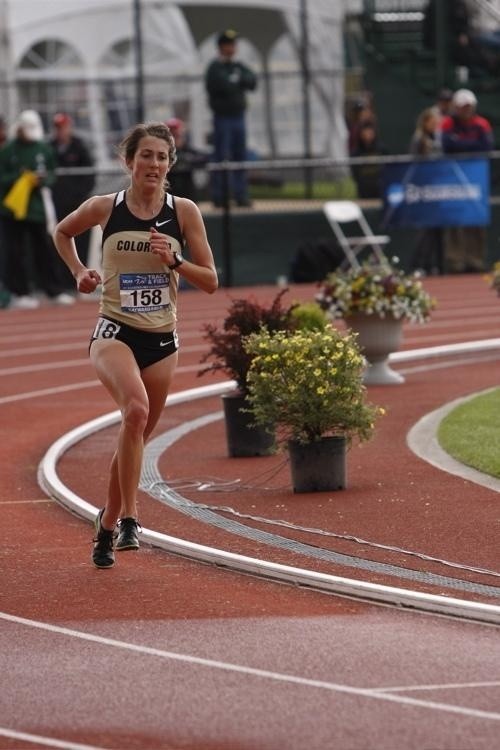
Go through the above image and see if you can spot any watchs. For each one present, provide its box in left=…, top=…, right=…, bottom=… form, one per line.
left=166, top=250, right=186, bottom=270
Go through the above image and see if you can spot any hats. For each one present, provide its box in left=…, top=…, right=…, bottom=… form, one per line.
left=19, top=111, right=44, bottom=141
left=217, top=29, right=239, bottom=44
left=455, top=89, right=476, bottom=109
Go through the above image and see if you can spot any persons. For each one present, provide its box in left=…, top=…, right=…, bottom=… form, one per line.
left=0, top=108, right=97, bottom=309
left=54, top=120, right=221, bottom=567
left=204, top=26, right=257, bottom=208
left=159, top=118, right=218, bottom=290
left=346, top=15, right=500, bottom=279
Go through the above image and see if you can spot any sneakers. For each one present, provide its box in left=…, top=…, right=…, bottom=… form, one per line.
left=50, top=293, right=74, bottom=305
left=11, top=294, right=40, bottom=310
left=91, top=508, right=115, bottom=569
left=115, top=517, right=142, bottom=551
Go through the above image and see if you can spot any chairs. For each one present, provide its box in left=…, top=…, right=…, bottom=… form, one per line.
left=321, top=200, right=391, bottom=273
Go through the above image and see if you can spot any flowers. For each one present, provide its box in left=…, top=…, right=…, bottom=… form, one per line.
left=314, top=255, right=440, bottom=328
left=236, top=322, right=392, bottom=452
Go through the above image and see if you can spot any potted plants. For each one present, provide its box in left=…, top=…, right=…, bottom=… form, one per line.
left=198, top=286, right=302, bottom=457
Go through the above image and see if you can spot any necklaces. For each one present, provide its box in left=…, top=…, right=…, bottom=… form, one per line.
left=133, top=196, right=162, bottom=216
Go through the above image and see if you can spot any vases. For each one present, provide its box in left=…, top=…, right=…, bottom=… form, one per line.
left=287, top=436, right=348, bottom=494
left=344, top=314, right=406, bottom=385
